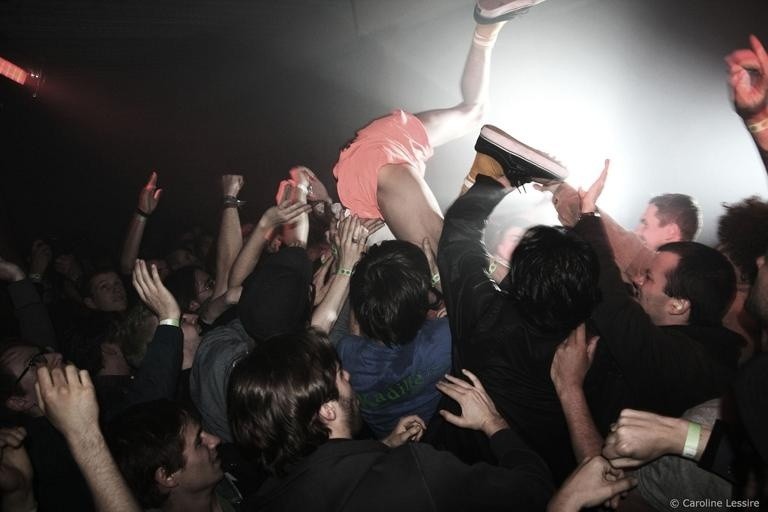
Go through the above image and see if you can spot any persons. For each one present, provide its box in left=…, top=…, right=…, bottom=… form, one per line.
left=327, top=2, right=568, bottom=257
left=0, top=32, right=767, bottom=511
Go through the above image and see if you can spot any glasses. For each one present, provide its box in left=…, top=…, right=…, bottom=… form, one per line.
left=183, top=274, right=218, bottom=303
left=7, top=338, right=56, bottom=402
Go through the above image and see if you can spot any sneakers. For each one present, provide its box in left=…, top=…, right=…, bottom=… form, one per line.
left=472, top=0, right=548, bottom=25
left=475, top=124, right=570, bottom=188
